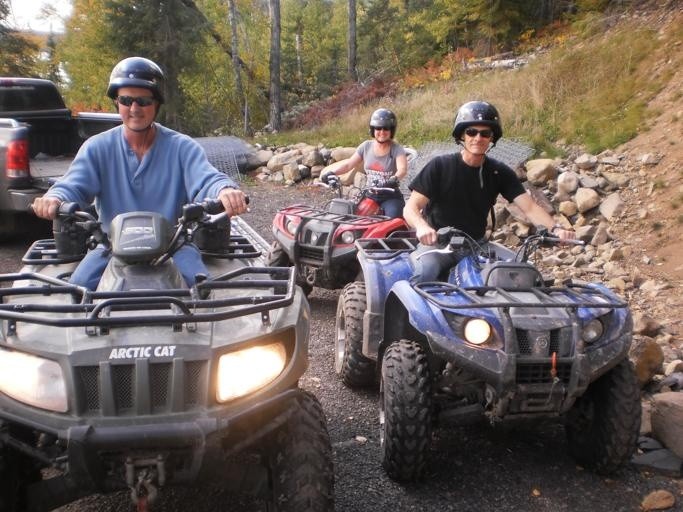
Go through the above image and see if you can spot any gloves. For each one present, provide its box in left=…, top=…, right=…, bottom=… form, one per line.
left=370, top=175, right=399, bottom=188
left=319, top=169, right=336, bottom=182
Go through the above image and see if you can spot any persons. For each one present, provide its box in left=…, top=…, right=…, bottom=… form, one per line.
left=320, top=107, right=411, bottom=218
left=401, top=100, right=578, bottom=291
left=31, top=55, right=248, bottom=295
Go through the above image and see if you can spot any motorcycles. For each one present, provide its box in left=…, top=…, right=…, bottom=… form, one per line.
left=267, top=174, right=419, bottom=296
left=335, top=226, right=640, bottom=485
left=0, top=195, right=335, bottom=511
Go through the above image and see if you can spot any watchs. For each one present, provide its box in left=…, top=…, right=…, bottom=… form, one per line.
left=551, top=223, right=563, bottom=233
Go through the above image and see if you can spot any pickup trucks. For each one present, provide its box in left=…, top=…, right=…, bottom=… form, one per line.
left=0, top=76, right=123, bottom=242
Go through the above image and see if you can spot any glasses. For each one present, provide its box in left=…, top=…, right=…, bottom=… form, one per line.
left=464, top=127, right=494, bottom=139
left=115, top=94, right=156, bottom=107
left=372, top=126, right=391, bottom=133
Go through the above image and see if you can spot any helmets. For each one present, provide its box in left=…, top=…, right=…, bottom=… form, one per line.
left=107, top=56, right=166, bottom=103
left=368, top=107, right=398, bottom=139
left=452, top=99, right=504, bottom=141
